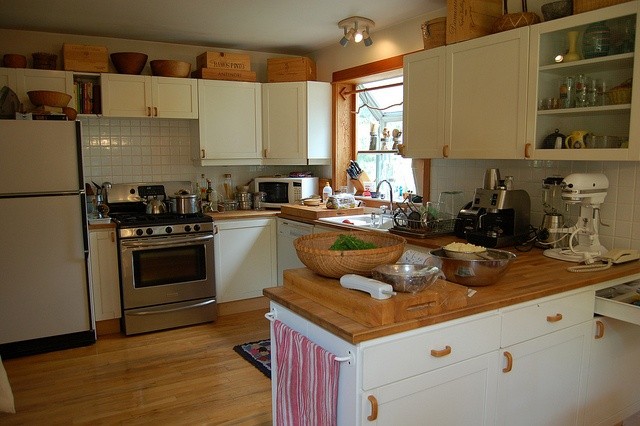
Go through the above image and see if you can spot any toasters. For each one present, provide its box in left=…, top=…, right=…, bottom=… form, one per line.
left=454, top=201, right=486, bottom=238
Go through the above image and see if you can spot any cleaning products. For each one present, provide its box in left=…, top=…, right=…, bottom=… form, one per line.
left=398, top=185, right=405, bottom=197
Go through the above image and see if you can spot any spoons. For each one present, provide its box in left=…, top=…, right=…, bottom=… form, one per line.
left=100, top=182, right=112, bottom=195
left=404, top=202, right=420, bottom=228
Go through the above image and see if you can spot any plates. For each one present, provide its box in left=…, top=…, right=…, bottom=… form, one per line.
left=304, top=199, right=322, bottom=205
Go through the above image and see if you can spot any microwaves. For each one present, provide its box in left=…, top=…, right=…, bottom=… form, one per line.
left=254, top=177, right=319, bottom=208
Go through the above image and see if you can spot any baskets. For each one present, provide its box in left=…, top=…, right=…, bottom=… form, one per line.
left=381, top=213, right=457, bottom=233
left=493, top=10, right=540, bottom=32
left=293, top=231, right=407, bottom=279
left=607, top=88, right=631, bottom=104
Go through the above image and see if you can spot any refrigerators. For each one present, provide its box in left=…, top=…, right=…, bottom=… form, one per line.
left=0, top=120, right=96, bottom=358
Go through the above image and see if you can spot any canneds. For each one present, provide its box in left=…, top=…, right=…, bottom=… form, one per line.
left=558, top=79, right=574, bottom=107
left=253, top=192, right=267, bottom=210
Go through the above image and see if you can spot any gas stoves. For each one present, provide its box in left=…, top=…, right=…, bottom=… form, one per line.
left=108, top=211, right=214, bottom=227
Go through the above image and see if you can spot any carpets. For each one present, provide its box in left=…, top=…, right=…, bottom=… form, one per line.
left=232, top=336, right=272, bottom=377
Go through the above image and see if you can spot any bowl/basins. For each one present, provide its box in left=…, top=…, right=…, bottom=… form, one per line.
left=63, top=107, right=77, bottom=121
left=372, top=263, right=436, bottom=293
left=252, top=192, right=267, bottom=210
left=421, top=212, right=436, bottom=233
left=585, top=136, right=622, bottom=148
left=428, top=248, right=516, bottom=286
left=224, top=201, right=239, bottom=210
left=370, top=192, right=381, bottom=198
left=235, top=192, right=252, bottom=209
left=5, top=54, right=26, bottom=68
left=236, top=185, right=249, bottom=193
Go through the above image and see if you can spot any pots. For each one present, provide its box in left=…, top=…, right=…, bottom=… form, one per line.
left=168, top=191, right=200, bottom=215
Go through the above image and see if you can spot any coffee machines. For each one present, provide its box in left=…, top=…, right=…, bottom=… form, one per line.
left=466, top=168, right=531, bottom=249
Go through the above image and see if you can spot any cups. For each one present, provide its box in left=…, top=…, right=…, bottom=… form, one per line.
left=565, top=131, right=589, bottom=148
left=545, top=74, right=611, bottom=109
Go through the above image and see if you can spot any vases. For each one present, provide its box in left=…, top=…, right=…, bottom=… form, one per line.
left=2, top=53, right=27, bottom=68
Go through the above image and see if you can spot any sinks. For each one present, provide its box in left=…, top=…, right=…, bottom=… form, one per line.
left=323, top=214, right=387, bottom=225
left=363, top=219, right=404, bottom=230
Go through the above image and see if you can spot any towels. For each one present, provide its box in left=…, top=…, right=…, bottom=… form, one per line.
left=265, top=320, right=338, bottom=425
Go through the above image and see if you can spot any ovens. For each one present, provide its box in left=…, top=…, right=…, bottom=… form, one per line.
left=117, top=222, right=217, bottom=336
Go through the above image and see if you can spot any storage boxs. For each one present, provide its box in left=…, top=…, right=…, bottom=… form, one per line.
left=61, top=43, right=110, bottom=72
left=265, top=56, right=318, bottom=82
left=446, top=1, right=504, bottom=44
left=195, top=51, right=254, bottom=69
left=190, top=67, right=258, bottom=82
left=420, top=16, right=447, bottom=49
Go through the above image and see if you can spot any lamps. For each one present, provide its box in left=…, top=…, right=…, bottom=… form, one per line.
left=337, top=15, right=375, bottom=48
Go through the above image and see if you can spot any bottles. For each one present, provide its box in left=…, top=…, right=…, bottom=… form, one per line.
left=201, top=188, right=207, bottom=203
left=224, top=174, right=233, bottom=200
left=195, top=183, right=201, bottom=210
left=323, top=182, right=333, bottom=204
left=207, top=180, right=214, bottom=202
left=218, top=201, right=225, bottom=212
left=200, top=174, right=207, bottom=188
left=326, top=196, right=338, bottom=209
left=211, top=188, right=218, bottom=211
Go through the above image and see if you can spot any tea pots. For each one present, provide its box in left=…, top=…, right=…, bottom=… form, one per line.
left=543, top=129, right=567, bottom=148
left=140, top=191, right=168, bottom=215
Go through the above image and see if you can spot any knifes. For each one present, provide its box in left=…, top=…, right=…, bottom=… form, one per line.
left=346, top=160, right=361, bottom=180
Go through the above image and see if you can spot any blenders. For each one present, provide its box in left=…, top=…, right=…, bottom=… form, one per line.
left=535, top=176, right=573, bottom=248
left=543, top=173, right=609, bottom=263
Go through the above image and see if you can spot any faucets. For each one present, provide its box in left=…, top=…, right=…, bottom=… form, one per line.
left=374, top=179, right=393, bottom=211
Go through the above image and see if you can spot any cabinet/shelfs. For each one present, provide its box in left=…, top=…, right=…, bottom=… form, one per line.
left=190, top=79, right=263, bottom=166
left=213, top=218, right=276, bottom=304
left=275, top=217, right=314, bottom=286
left=263, top=80, right=332, bottom=166
left=403, top=45, right=448, bottom=158
left=524, top=0, right=639, bottom=161
left=449, top=24, right=530, bottom=159
left=352, top=314, right=500, bottom=426
left=89, top=227, right=122, bottom=322
left=594, top=279, right=640, bottom=426
left=102, top=76, right=197, bottom=119
left=501, top=288, right=595, bottom=426
left=0, top=68, right=74, bottom=111
